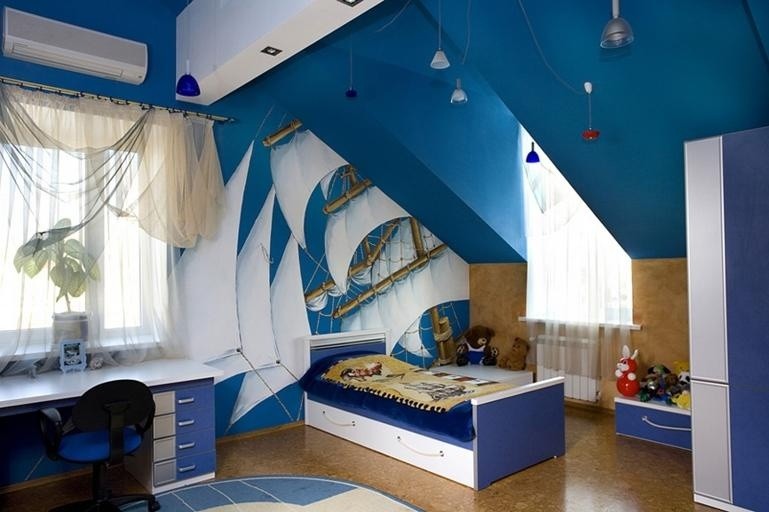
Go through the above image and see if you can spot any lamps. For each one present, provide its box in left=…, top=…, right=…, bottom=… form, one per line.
left=526, top=142, right=540, bottom=164
left=176, top=59, right=201, bottom=97
left=599, top=1, right=635, bottom=49
left=450, top=78, right=467, bottom=104
left=430, top=51, right=450, bottom=69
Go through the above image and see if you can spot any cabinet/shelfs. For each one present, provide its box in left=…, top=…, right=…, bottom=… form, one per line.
left=684, top=124, right=768, bottom=511
left=614, top=393, right=693, bottom=452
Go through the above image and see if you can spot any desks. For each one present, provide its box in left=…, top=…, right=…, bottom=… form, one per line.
left=1, top=357, right=223, bottom=496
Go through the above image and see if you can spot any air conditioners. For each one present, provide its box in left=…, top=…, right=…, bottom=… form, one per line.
left=1, top=6, right=148, bottom=86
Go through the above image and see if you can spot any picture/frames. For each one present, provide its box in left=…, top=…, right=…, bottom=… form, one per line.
left=58, top=341, right=88, bottom=373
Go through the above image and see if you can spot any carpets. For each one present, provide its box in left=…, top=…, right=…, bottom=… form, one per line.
left=117, top=472, right=422, bottom=512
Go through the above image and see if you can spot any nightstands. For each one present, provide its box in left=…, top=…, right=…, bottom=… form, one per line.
left=430, top=359, right=534, bottom=388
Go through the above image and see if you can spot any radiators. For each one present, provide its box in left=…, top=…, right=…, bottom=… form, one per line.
left=536, top=335, right=603, bottom=403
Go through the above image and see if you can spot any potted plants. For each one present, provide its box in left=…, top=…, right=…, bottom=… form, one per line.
left=13, top=218, right=98, bottom=344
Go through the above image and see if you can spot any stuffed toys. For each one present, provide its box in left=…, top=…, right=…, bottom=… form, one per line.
left=641, top=362, right=691, bottom=409
left=500, top=336, right=531, bottom=371
left=456, top=326, right=500, bottom=366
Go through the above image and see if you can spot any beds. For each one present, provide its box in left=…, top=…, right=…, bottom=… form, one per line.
left=303, top=331, right=567, bottom=493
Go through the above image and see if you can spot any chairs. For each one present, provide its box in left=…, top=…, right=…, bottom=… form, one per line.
left=40, top=380, right=162, bottom=511
